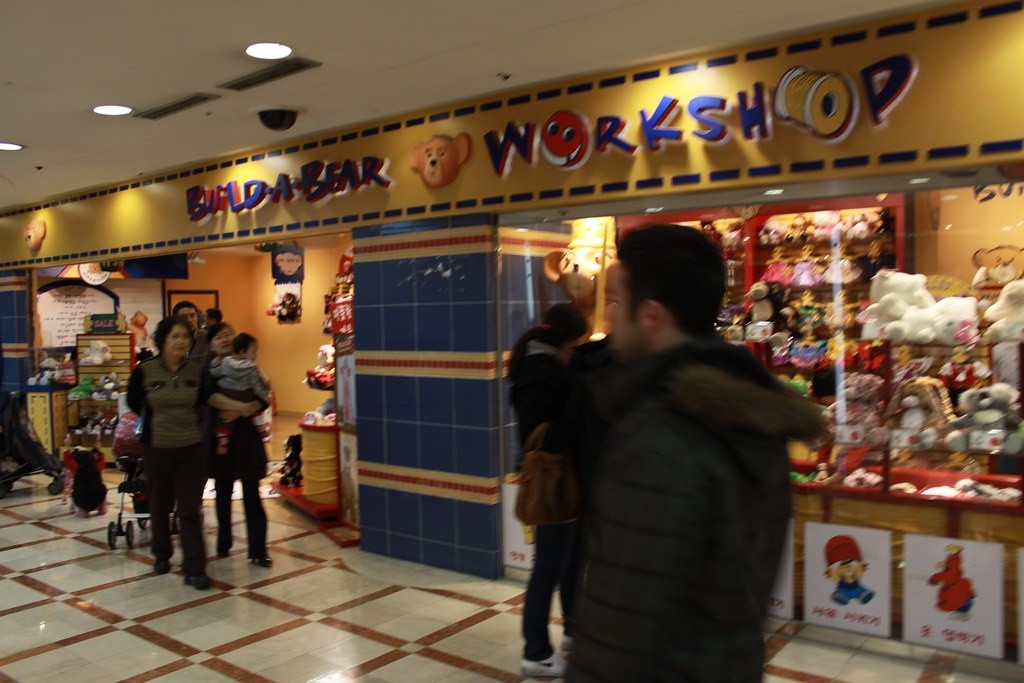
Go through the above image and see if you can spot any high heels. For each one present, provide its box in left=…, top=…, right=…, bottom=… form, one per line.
left=252, top=555, right=272, bottom=567
left=219, top=547, right=228, bottom=556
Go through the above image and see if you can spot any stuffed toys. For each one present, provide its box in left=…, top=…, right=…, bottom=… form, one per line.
left=307, top=344, right=336, bottom=388
left=28, top=340, right=123, bottom=435
left=734, top=209, right=1024, bottom=473
left=279, top=433, right=303, bottom=488
left=275, top=293, right=302, bottom=316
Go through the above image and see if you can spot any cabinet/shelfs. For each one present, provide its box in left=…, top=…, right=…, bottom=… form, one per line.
left=58, top=334, right=135, bottom=463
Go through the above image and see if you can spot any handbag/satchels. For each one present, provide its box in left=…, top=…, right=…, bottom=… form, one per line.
left=516, top=421, right=582, bottom=525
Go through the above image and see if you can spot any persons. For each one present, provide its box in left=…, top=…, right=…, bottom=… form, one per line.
left=61, top=448, right=108, bottom=519
left=172, top=299, right=274, bottom=569
left=507, top=303, right=643, bottom=683
left=127, top=314, right=214, bottom=591
left=565, top=222, right=829, bottom=683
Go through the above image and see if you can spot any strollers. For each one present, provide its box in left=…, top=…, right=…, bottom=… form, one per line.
left=0, top=390, right=63, bottom=500
left=108, top=455, right=182, bottom=551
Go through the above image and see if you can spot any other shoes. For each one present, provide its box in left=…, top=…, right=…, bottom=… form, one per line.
left=562, top=634, right=575, bottom=650
left=261, top=429, right=272, bottom=443
left=521, top=653, right=569, bottom=677
left=154, top=558, right=169, bottom=573
left=216, top=444, right=228, bottom=455
left=185, top=574, right=208, bottom=589
